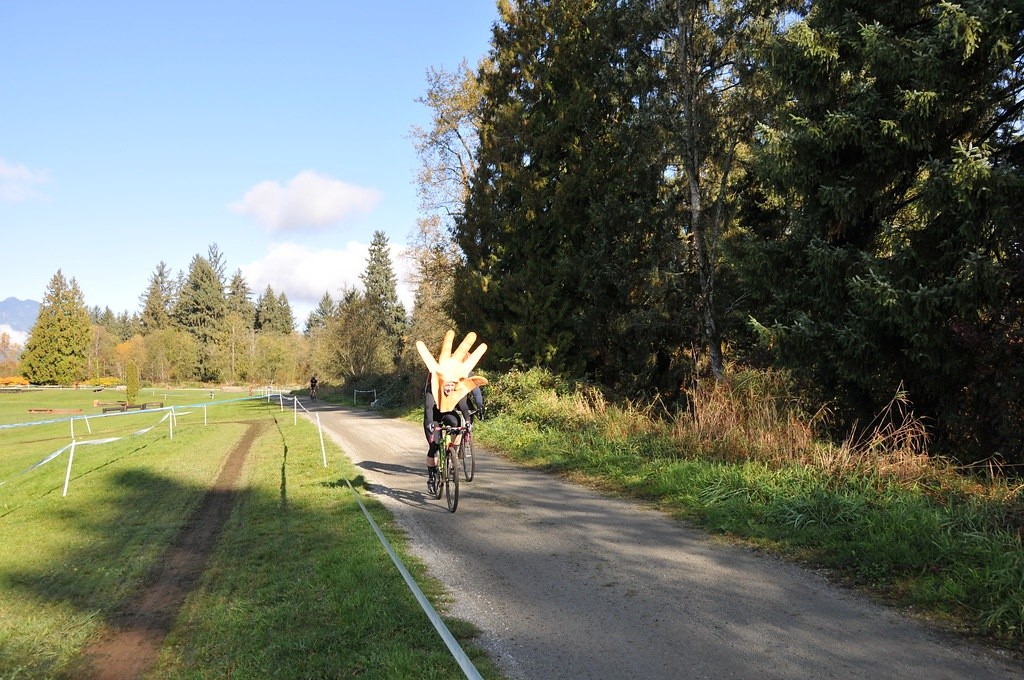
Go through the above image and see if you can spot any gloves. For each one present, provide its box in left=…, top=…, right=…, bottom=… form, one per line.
left=477, top=406, right=485, bottom=414
left=427, top=422, right=440, bottom=433
left=465, top=423, right=473, bottom=432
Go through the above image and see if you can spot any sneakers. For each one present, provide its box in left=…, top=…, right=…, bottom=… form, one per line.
left=451, top=459, right=461, bottom=469
left=427, top=481, right=436, bottom=493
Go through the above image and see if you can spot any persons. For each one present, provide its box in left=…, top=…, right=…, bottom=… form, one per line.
left=424, top=371, right=471, bottom=487
left=310, top=377, right=317, bottom=395
left=453, top=353, right=483, bottom=423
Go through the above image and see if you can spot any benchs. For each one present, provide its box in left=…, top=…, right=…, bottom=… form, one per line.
left=103, top=401, right=163, bottom=414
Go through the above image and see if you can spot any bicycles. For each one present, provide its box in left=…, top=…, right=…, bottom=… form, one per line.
left=426, top=422, right=471, bottom=512
left=453, top=401, right=493, bottom=482
left=310, top=387, right=317, bottom=403
